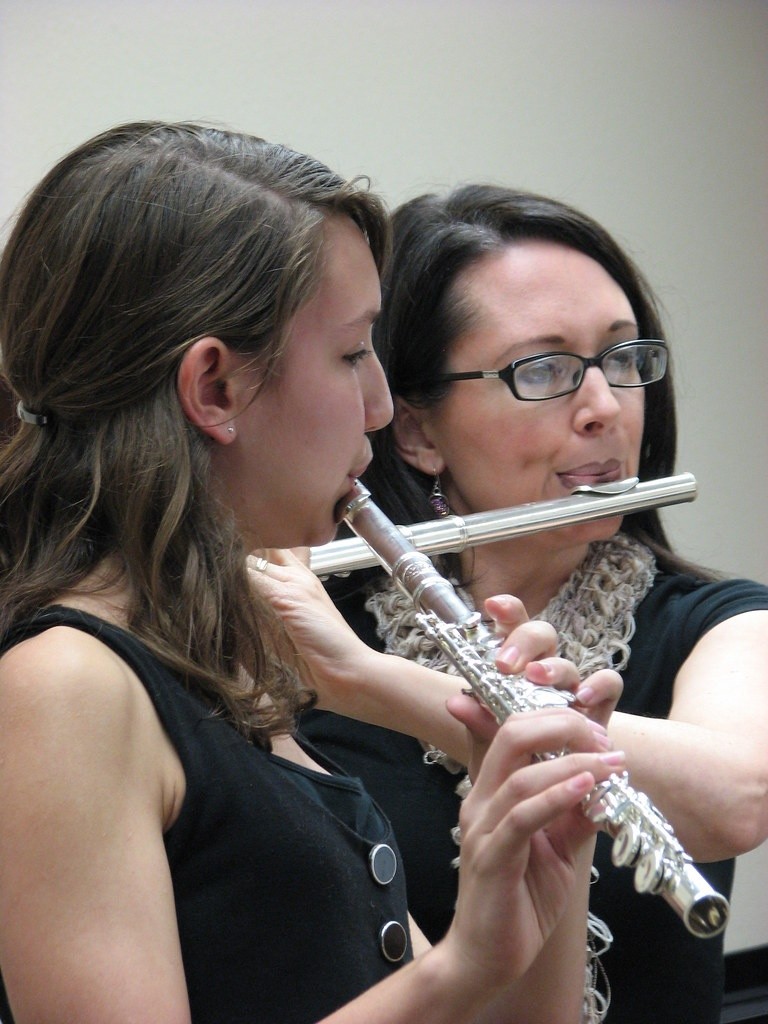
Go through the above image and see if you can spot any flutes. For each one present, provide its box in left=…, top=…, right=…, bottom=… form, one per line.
left=329, top=461, right=732, bottom=937
left=279, top=473, right=698, bottom=577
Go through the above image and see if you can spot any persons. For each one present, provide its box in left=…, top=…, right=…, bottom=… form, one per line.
left=282, top=182, right=767, bottom=1024
left=0, top=118, right=623, bottom=1024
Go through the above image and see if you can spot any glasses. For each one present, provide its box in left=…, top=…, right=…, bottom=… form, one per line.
left=441, top=339, right=669, bottom=402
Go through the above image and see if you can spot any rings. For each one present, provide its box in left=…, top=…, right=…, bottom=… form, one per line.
left=255, top=558, right=271, bottom=571
